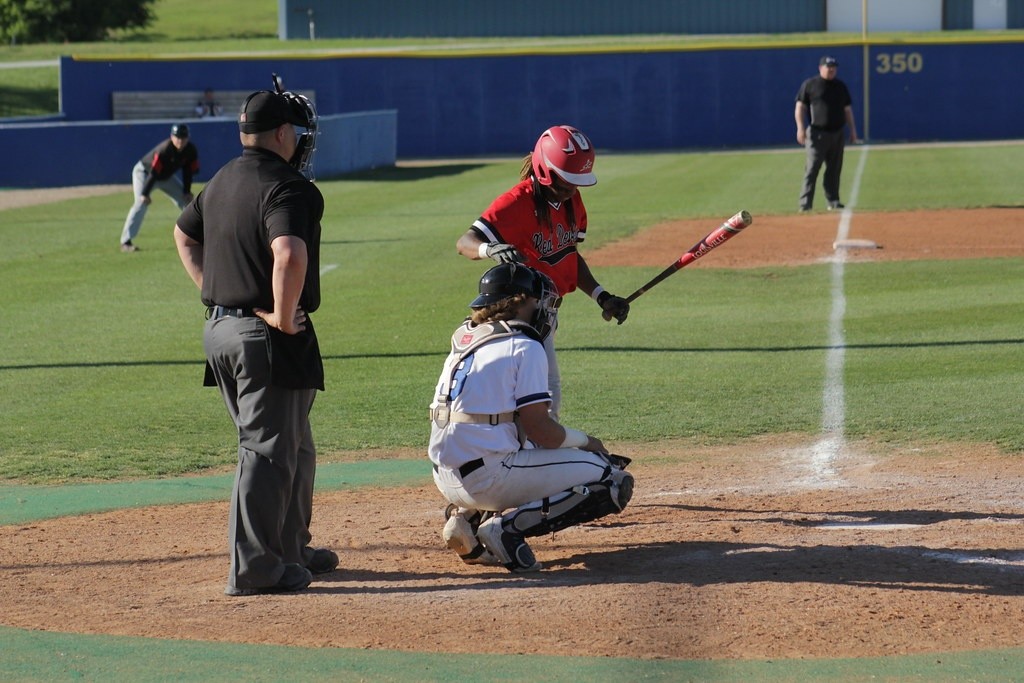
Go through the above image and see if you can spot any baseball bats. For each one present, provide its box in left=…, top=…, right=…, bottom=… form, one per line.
left=602, top=210, right=752, bottom=321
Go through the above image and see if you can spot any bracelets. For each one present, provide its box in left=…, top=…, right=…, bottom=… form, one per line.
left=478, top=242, right=490, bottom=258
left=591, top=285, right=605, bottom=301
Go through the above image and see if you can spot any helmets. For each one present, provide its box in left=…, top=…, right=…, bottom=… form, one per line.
left=471, top=261, right=547, bottom=307
left=531, top=125, right=597, bottom=186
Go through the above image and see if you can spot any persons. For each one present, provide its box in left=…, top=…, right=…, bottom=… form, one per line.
left=194, top=88, right=223, bottom=118
left=794, top=55, right=859, bottom=212
left=120, top=124, right=199, bottom=252
left=429, top=261, right=636, bottom=573
left=173, top=72, right=339, bottom=597
left=453, top=124, right=630, bottom=423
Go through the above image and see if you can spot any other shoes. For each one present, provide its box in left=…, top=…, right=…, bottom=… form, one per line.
left=121, top=240, right=139, bottom=253
left=827, top=200, right=844, bottom=211
left=799, top=206, right=813, bottom=214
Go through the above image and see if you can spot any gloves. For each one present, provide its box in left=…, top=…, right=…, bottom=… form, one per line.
left=478, top=239, right=522, bottom=266
left=592, top=286, right=630, bottom=325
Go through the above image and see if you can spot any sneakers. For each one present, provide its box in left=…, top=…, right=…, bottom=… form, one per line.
left=478, top=516, right=542, bottom=572
left=225, top=562, right=312, bottom=595
left=306, top=547, right=339, bottom=575
left=450, top=518, right=499, bottom=565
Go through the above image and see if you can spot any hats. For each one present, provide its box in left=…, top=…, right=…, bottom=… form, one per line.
left=238, top=89, right=296, bottom=135
left=171, top=124, right=189, bottom=138
left=819, top=56, right=838, bottom=67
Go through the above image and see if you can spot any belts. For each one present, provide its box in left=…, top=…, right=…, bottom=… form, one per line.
left=433, top=457, right=485, bottom=479
left=209, top=306, right=274, bottom=318
